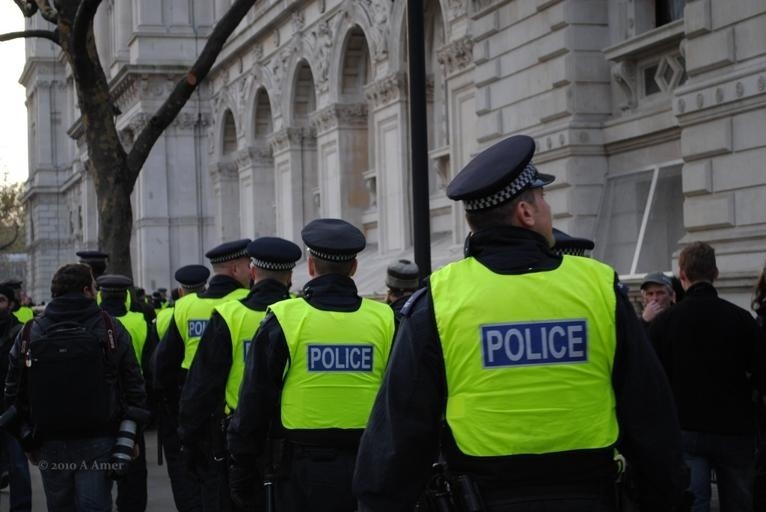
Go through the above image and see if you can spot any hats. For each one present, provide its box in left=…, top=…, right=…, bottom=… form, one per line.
left=638, top=273, right=675, bottom=297
left=248, top=237, right=302, bottom=270
left=446, top=135, right=555, bottom=210
left=552, top=227, right=595, bottom=258
left=174, top=265, right=211, bottom=289
left=385, top=260, right=420, bottom=289
left=76, top=250, right=110, bottom=263
left=97, top=274, right=133, bottom=293
left=206, top=238, right=251, bottom=264
left=301, top=218, right=366, bottom=263
left=0, top=275, right=23, bottom=287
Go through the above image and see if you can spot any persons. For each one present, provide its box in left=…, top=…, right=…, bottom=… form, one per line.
left=146, top=238, right=254, bottom=512
left=636, top=241, right=765, bottom=512
left=75, top=250, right=133, bottom=311
left=1, top=284, right=33, bottom=512
left=134, top=283, right=183, bottom=325
left=175, top=237, right=302, bottom=512
left=350, top=134, right=695, bottom=512
left=549, top=225, right=595, bottom=258
left=95, top=274, right=150, bottom=377
left=145, top=264, right=210, bottom=404
left=0, top=264, right=147, bottom=512
left=223, top=217, right=396, bottom=512
left=0, top=276, right=46, bottom=325
left=385, top=256, right=421, bottom=309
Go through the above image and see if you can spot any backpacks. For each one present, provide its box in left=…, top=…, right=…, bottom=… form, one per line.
left=25, top=311, right=121, bottom=454
